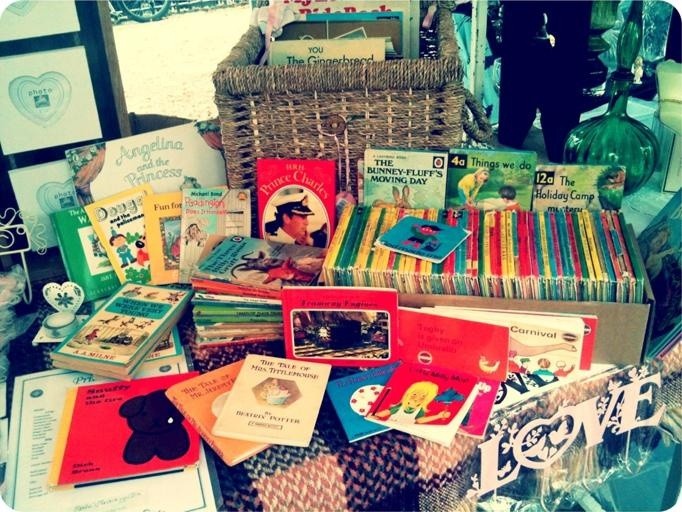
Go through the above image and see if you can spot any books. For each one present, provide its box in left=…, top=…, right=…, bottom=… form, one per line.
left=48, top=145, right=647, bottom=490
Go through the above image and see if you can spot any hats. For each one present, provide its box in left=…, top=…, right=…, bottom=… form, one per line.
left=271, top=193, right=316, bottom=215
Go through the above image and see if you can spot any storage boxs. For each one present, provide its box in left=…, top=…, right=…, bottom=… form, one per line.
left=316, top=224, right=656, bottom=364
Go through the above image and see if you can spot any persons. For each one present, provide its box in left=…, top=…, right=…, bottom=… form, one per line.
left=498, top=1, right=592, bottom=163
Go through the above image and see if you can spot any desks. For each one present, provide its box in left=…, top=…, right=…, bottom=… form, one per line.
left=0, top=276, right=682, bottom=512
